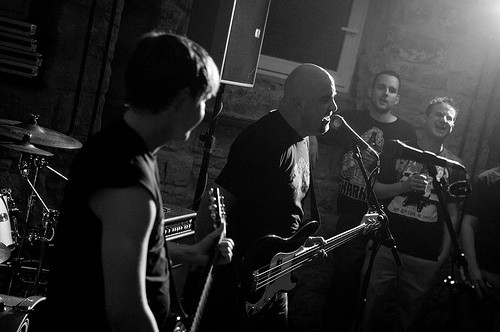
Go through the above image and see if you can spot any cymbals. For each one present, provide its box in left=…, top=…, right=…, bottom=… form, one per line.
left=0, top=118, right=83, bottom=156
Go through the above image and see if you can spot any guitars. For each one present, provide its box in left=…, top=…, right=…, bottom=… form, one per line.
left=237, top=209, right=384, bottom=318
left=163, top=187, right=228, bottom=332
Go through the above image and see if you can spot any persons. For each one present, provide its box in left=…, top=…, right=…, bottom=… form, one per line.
left=309, top=69, right=419, bottom=332
left=461, top=166, right=500, bottom=299
left=194, top=63, right=328, bottom=331
left=47, top=33, right=235, bottom=332
left=364, top=96, right=465, bottom=332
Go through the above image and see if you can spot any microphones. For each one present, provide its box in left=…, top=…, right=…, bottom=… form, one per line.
left=383, top=140, right=465, bottom=170
left=329, top=114, right=379, bottom=158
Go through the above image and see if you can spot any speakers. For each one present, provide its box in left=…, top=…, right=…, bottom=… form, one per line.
left=187, top=0, right=272, bottom=88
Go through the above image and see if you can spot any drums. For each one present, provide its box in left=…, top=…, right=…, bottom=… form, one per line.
left=0, top=192, right=17, bottom=266
